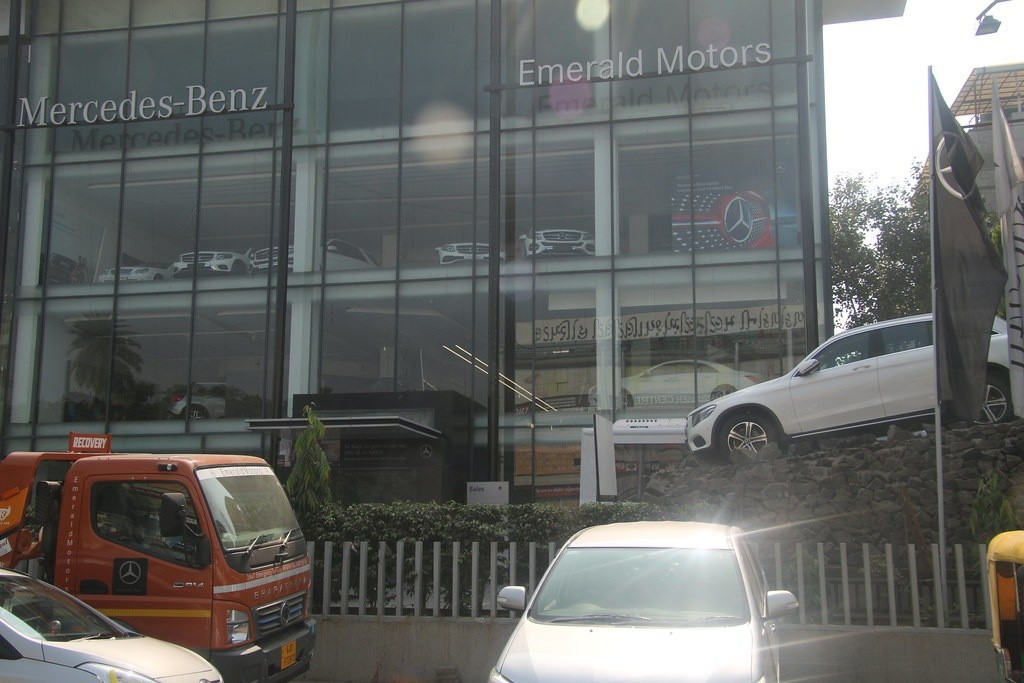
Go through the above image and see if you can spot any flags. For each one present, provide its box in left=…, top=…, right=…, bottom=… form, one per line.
left=930, top=70, right=1010, bottom=425
left=990, top=88, right=1024, bottom=418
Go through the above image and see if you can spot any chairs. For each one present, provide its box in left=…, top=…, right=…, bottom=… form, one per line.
left=99, top=487, right=158, bottom=538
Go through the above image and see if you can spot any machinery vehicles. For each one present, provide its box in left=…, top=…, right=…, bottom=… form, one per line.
left=1, top=434, right=318, bottom=682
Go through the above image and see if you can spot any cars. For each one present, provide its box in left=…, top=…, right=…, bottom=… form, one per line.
left=249, top=232, right=380, bottom=273
left=684, top=305, right=1011, bottom=468
left=1, top=568, right=228, bottom=683
left=96, top=259, right=173, bottom=280
left=516, top=224, right=597, bottom=260
left=585, top=357, right=762, bottom=408
left=173, top=249, right=253, bottom=274
left=168, top=378, right=231, bottom=418
left=488, top=520, right=800, bottom=683
left=425, top=239, right=506, bottom=267
